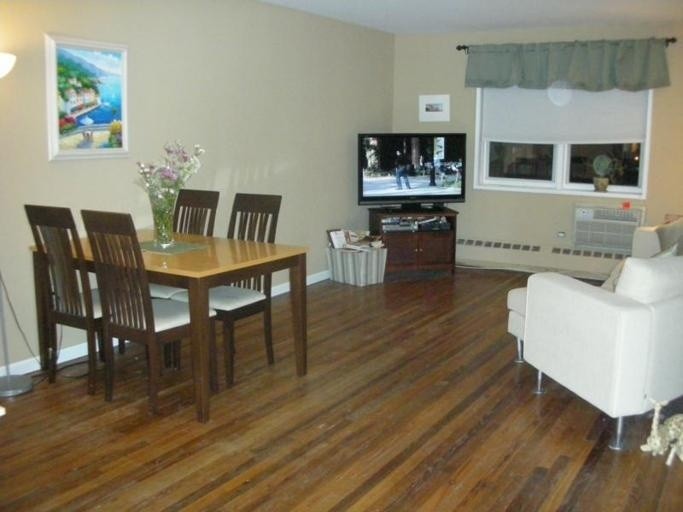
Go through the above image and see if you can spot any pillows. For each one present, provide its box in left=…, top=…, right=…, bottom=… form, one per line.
left=616, top=256, right=682, bottom=303
left=657, top=216, right=683, bottom=256
left=649, top=243, right=680, bottom=258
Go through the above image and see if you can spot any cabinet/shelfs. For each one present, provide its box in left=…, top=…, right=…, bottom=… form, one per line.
left=369, top=205, right=458, bottom=272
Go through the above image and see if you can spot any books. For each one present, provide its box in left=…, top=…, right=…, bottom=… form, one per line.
left=326, top=228, right=384, bottom=250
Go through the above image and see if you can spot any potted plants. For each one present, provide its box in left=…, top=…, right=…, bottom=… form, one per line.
left=592, top=155, right=613, bottom=192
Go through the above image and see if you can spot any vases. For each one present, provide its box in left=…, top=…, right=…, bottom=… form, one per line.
left=143, top=188, right=182, bottom=247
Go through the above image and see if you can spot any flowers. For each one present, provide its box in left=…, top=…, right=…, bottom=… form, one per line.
left=140, top=143, right=202, bottom=239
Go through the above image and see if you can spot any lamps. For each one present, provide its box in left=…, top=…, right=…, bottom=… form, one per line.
left=2, top=53, right=31, bottom=401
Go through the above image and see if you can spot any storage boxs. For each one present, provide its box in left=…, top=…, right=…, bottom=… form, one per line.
left=328, top=247, right=387, bottom=286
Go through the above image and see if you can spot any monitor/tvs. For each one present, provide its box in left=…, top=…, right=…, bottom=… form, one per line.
left=358, top=133, right=466, bottom=213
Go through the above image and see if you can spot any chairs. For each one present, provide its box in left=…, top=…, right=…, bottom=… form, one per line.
left=134, top=190, right=224, bottom=368
left=81, top=211, right=217, bottom=424
left=193, top=194, right=282, bottom=385
left=24, top=202, right=147, bottom=397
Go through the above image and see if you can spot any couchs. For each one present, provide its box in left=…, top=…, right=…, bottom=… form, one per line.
left=507, top=224, right=683, bottom=452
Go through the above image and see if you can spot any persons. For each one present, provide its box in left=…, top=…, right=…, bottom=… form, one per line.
left=395, top=150, right=411, bottom=190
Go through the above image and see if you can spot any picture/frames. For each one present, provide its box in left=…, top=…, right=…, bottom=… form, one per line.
left=45, top=31, right=130, bottom=162
